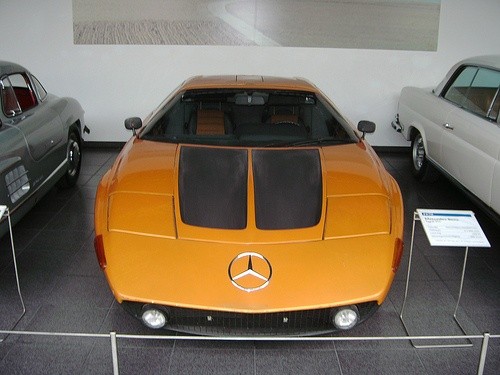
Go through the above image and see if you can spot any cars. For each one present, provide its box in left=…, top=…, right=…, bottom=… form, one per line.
left=392, top=54, right=500, bottom=233
left=0, top=61, right=91, bottom=240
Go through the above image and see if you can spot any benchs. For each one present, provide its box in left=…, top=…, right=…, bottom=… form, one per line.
left=4, top=86, right=35, bottom=115
left=445, top=86, right=500, bottom=119
left=179, top=101, right=301, bottom=132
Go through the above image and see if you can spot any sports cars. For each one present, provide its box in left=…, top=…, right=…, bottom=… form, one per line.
left=93, top=75, right=404, bottom=337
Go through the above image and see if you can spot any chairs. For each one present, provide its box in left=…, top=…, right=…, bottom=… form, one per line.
left=265, top=93, right=306, bottom=127
left=187, top=94, right=235, bottom=138
